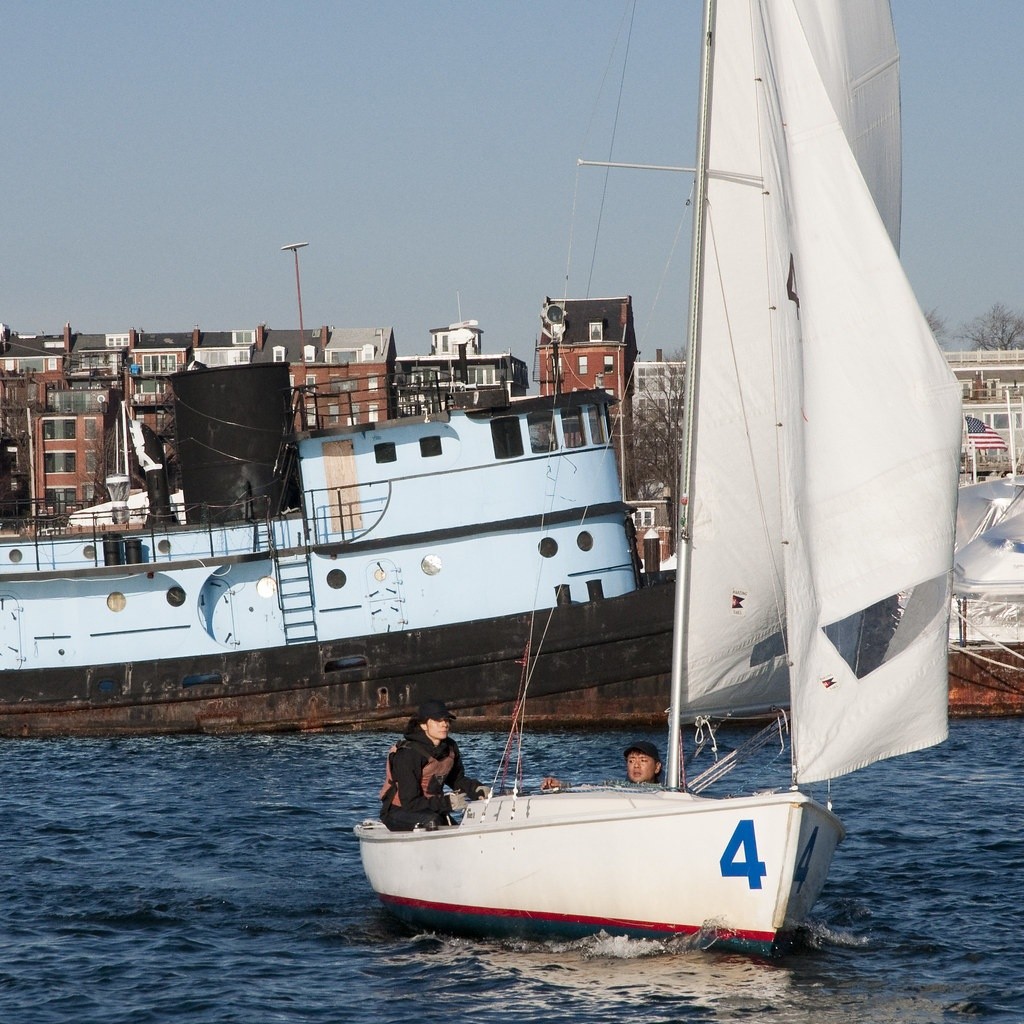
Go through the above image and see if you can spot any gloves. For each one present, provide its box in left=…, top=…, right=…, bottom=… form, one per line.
left=475, top=785, right=491, bottom=799
left=450, top=788, right=467, bottom=811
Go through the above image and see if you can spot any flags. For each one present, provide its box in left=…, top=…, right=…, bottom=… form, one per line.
left=965, top=416, right=1008, bottom=453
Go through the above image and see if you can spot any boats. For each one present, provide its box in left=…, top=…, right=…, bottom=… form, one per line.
left=0, top=299, right=677, bottom=736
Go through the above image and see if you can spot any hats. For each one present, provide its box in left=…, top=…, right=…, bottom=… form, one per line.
left=417, top=699, right=456, bottom=724
left=623, top=741, right=660, bottom=761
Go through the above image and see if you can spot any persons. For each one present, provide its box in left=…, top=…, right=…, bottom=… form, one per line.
left=540, top=741, right=663, bottom=791
left=378, top=701, right=492, bottom=831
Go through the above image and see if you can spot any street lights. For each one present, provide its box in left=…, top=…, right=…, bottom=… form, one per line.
left=284, top=242, right=308, bottom=392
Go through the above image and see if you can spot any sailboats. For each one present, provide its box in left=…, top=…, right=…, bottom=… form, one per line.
left=349, top=0, right=964, bottom=961
